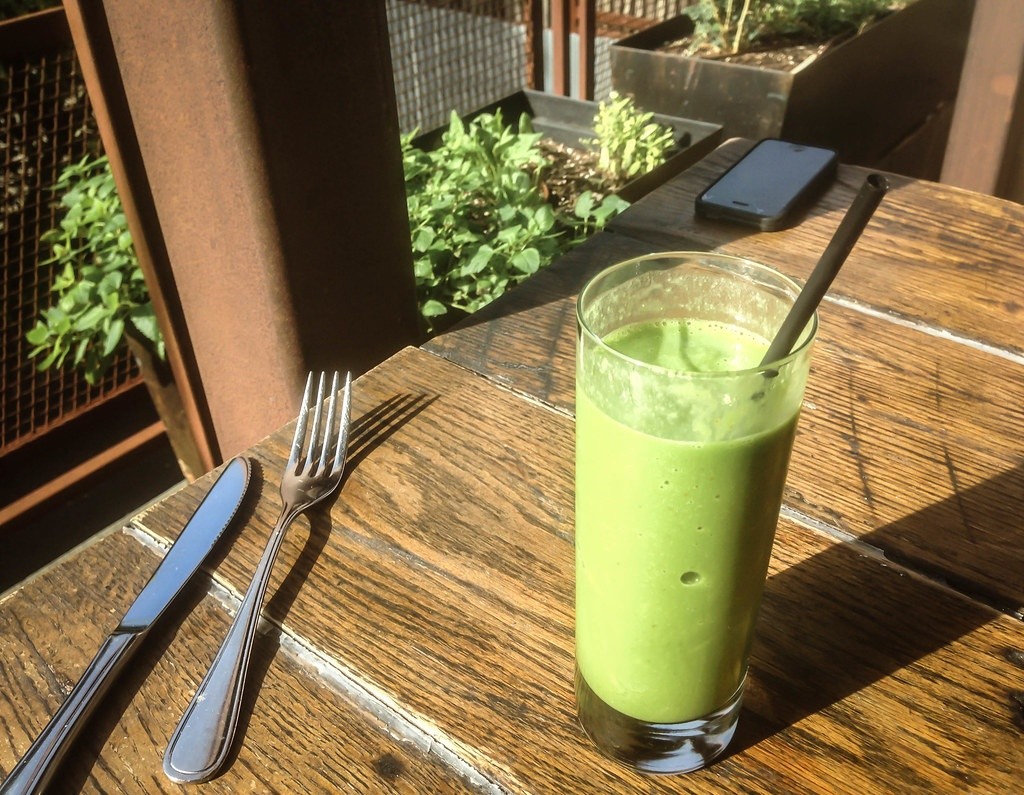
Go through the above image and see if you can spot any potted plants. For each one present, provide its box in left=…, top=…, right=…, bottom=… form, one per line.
left=27, top=87, right=723, bottom=484
left=609, top=1, right=966, bottom=159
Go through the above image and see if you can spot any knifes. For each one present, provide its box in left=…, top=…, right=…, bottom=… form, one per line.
left=0, top=456, right=251, bottom=795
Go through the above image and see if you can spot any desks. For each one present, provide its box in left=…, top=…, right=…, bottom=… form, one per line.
left=0, top=135, right=1024, bottom=795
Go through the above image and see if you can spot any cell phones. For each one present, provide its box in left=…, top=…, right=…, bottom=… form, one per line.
left=696, top=139, right=839, bottom=231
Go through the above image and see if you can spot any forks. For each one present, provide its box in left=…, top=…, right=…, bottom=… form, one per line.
left=161, top=369, right=351, bottom=784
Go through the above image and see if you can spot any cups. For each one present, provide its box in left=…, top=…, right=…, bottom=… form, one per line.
left=570, top=253, right=818, bottom=776
